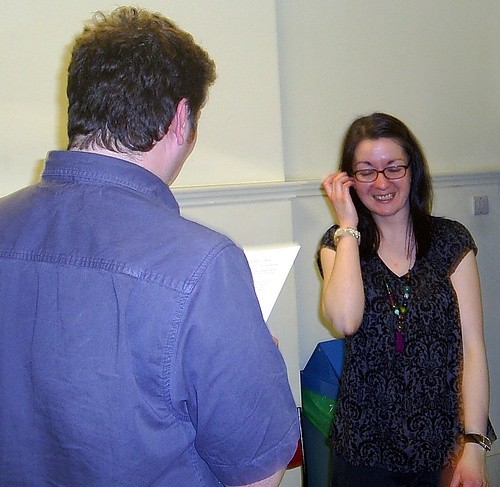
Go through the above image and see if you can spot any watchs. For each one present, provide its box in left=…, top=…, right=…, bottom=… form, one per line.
left=464, top=433, right=492, bottom=451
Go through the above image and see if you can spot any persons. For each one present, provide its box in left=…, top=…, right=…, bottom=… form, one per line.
left=0, top=8, right=301, bottom=487
left=316, top=113, right=497, bottom=487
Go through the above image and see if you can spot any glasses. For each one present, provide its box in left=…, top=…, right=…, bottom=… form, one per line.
left=351, top=157, right=411, bottom=183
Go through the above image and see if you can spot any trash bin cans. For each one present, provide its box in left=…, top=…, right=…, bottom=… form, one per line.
left=296, top=336, right=344, bottom=484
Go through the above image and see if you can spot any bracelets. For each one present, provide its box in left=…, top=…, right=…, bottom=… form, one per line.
left=333, top=227, right=361, bottom=246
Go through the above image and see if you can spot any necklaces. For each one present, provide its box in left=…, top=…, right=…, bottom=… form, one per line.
left=374, top=212, right=413, bottom=353
